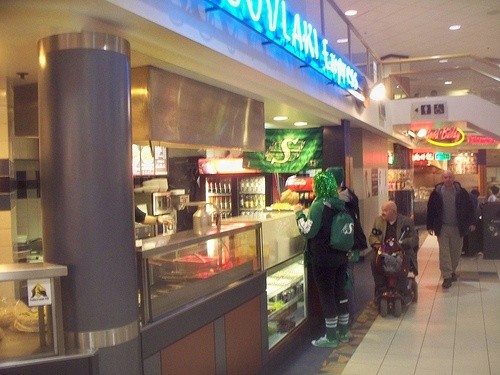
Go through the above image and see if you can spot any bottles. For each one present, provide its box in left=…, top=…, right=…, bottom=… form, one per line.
left=209, top=177, right=264, bottom=217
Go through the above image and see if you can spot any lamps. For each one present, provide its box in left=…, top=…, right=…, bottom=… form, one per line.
left=356, top=82, right=386, bottom=109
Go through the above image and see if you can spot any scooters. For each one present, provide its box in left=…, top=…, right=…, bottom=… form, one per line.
left=372, top=224, right=416, bottom=317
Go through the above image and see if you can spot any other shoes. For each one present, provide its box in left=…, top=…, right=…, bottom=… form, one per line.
left=452, top=273, right=458, bottom=281
left=442, top=278, right=452, bottom=288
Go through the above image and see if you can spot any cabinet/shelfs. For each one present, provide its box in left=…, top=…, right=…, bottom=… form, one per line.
left=263, top=257, right=307, bottom=353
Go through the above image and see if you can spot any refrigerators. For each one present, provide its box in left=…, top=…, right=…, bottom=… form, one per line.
left=198, top=155, right=286, bottom=222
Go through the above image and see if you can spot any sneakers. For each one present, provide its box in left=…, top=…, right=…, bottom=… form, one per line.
left=311, top=334, right=339, bottom=348
left=337, top=334, right=349, bottom=343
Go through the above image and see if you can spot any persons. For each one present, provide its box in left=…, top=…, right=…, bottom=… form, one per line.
left=369, top=201, right=418, bottom=307
left=135, top=206, right=173, bottom=225
left=325, top=167, right=360, bottom=329
left=461, top=184, right=500, bottom=256
left=426, top=170, right=476, bottom=289
left=294, top=166, right=349, bottom=348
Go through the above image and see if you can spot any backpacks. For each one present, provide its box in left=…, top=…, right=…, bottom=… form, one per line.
left=329, top=210, right=354, bottom=254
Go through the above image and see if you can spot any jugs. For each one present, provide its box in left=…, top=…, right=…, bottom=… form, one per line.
left=192, top=201, right=220, bottom=236
